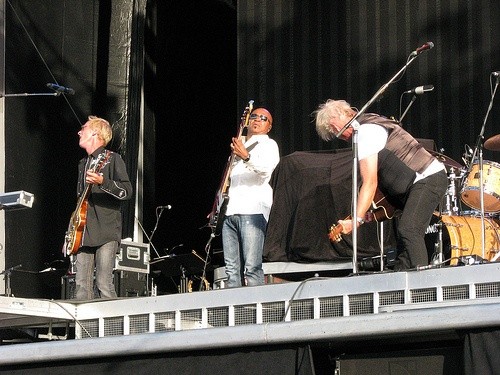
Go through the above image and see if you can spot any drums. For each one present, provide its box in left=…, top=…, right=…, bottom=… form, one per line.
left=459, top=159, right=500, bottom=213
left=423, top=211, right=500, bottom=267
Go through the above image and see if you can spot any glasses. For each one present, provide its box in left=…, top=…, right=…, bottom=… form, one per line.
left=250, top=114, right=269, bottom=123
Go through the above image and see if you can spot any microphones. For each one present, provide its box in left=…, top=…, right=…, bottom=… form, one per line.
left=492, top=71, right=500, bottom=76
left=412, top=41, right=434, bottom=56
left=158, top=205, right=171, bottom=209
left=47, top=84, right=75, bottom=95
left=467, top=145, right=474, bottom=156
left=405, top=85, right=435, bottom=94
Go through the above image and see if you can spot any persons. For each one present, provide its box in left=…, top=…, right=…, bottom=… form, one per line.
left=76, top=118, right=132, bottom=301
left=207, top=108, right=280, bottom=288
left=317, top=101, right=448, bottom=271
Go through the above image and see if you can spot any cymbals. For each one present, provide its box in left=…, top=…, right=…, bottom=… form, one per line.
left=426, top=149, right=463, bottom=170
left=483, top=134, right=500, bottom=152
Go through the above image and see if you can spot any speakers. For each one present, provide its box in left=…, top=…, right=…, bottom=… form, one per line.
left=61, top=271, right=148, bottom=301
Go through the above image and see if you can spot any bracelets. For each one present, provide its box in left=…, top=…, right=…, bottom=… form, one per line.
left=357, top=217, right=364, bottom=225
left=244, top=155, right=249, bottom=162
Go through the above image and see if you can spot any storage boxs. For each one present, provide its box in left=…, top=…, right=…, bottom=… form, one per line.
left=70, top=242, right=151, bottom=274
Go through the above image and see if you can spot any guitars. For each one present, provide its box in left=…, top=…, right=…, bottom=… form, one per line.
left=209, top=100, right=255, bottom=239
left=328, top=178, right=397, bottom=245
left=61, top=147, right=113, bottom=258
